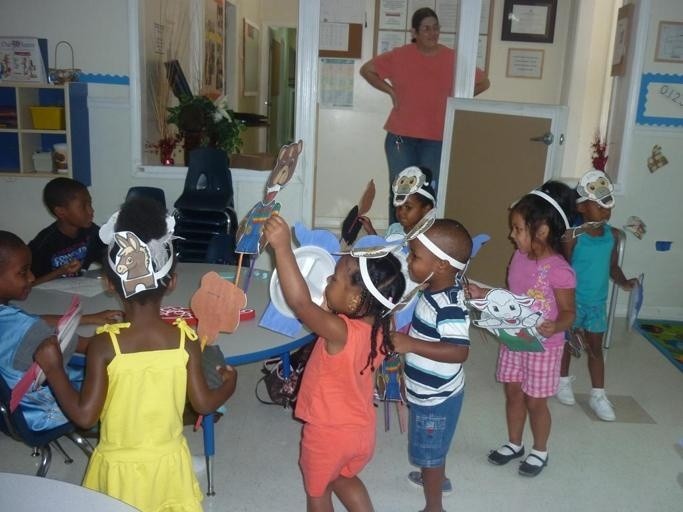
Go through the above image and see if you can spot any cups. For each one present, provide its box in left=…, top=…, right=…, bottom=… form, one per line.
left=53, top=143, right=67, bottom=173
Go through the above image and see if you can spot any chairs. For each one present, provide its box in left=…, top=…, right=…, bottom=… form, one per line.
left=0, top=374, right=94, bottom=477
left=171, top=149, right=238, bottom=264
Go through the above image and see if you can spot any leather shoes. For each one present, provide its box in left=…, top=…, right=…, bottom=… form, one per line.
left=488, top=445, right=525, bottom=466
left=518, top=452, right=549, bottom=476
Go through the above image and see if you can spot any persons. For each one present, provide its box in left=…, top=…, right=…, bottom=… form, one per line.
left=385, top=218, right=473, bottom=512
left=262, top=213, right=406, bottom=512
left=27, top=177, right=109, bottom=286
left=359, top=167, right=436, bottom=234
left=33, top=200, right=237, bottom=512
left=555, top=169, right=639, bottom=421
left=360, top=8, right=490, bottom=225
left=463, top=179, right=576, bottom=477
left=0, top=228, right=86, bottom=433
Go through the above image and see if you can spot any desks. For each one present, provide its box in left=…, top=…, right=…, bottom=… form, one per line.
left=0, top=472, right=142, bottom=511
left=7, top=264, right=331, bottom=497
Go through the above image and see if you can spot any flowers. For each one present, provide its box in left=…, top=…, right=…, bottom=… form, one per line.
left=165, top=94, right=243, bottom=153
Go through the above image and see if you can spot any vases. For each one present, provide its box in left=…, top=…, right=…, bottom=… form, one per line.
left=183, top=120, right=202, bottom=166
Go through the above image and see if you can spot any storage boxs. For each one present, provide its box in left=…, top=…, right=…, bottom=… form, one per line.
left=32, top=151, right=56, bottom=174
left=29, top=106, right=64, bottom=130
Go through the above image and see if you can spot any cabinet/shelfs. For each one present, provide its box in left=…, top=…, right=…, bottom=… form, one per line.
left=0, top=81, right=91, bottom=187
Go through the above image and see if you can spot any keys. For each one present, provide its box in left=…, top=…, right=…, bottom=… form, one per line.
left=395, top=136, right=404, bottom=152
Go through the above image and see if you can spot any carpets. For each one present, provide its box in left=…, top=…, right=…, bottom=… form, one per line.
left=634, top=320, right=683, bottom=374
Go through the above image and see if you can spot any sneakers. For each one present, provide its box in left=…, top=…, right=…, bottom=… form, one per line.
left=588, top=389, right=616, bottom=422
left=407, top=471, right=454, bottom=496
left=554, top=376, right=577, bottom=405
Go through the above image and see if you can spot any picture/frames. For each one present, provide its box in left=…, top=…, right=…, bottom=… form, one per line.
left=501, top=0, right=558, bottom=43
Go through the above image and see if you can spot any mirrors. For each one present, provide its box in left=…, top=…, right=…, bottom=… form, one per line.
left=243, top=18, right=261, bottom=97
